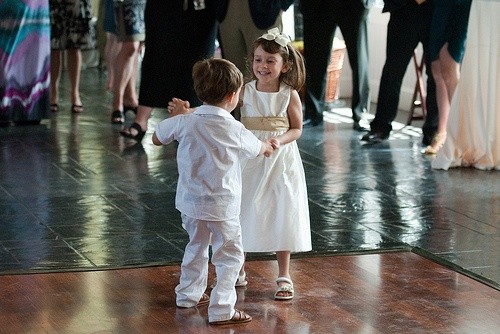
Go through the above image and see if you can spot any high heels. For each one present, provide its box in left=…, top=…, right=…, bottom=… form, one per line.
left=120, top=122, right=146, bottom=142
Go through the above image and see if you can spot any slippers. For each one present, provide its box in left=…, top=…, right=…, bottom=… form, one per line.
left=111, top=109, right=125, bottom=123
left=123, top=105, right=138, bottom=115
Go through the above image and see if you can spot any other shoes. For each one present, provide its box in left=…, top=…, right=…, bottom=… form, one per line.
left=301, top=116, right=313, bottom=127
left=361, top=128, right=385, bottom=142
left=353, top=119, right=371, bottom=132
left=425, top=134, right=445, bottom=153
left=423, top=134, right=432, bottom=145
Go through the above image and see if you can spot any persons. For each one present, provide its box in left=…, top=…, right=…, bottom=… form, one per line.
left=0, top=0, right=500, bottom=173
left=152, top=57, right=274, bottom=325
left=167, top=28, right=312, bottom=301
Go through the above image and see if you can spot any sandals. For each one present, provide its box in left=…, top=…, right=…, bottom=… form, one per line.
left=198, top=294, right=210, bottom=304
left=72, top=103, right=84, bottom=114
left=210, top=271, right=248, bottom=289
left=50, top=103, right=59, bottom=112
left=210, top=309, right=252, bottom=324
left=274, top=277, right=294, bottom=300
